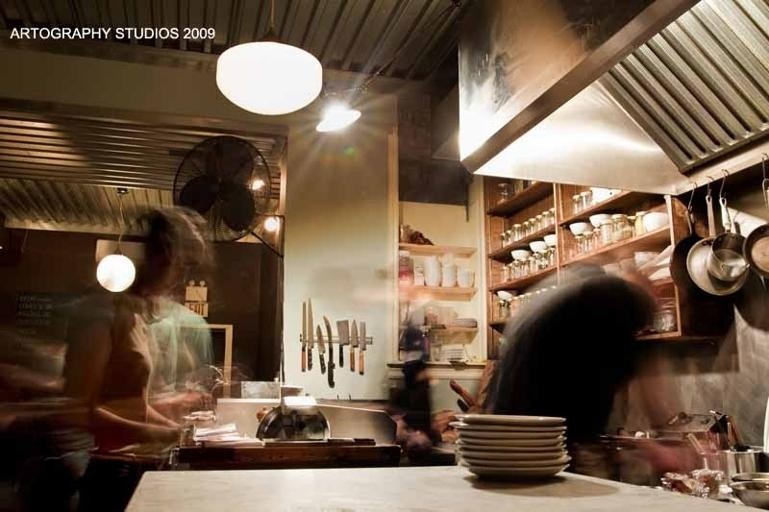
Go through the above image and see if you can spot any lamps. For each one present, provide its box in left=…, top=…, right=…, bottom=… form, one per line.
left=216, top=0, right=323, bottom=116
left=96, top=183, right=136, bottom=293
left=311, top=0, right=468, bottom=133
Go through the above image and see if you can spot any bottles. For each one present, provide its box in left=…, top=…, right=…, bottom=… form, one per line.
left=415, top=266, right=424, bottom=286
left=399, top=251, right=414, bottom=287
left=424, top=254, right=473, bottom=289
left=490, top=179, right=675, bottom=334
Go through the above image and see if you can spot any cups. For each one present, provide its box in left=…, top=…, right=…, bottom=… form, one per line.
left=697, top=448, right=763, bottom=475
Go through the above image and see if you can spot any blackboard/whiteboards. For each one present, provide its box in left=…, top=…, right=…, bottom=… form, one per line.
left=11, top=289, right=96, bottom=344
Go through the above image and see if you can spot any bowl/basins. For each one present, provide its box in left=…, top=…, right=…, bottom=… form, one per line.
left=730, top=471, right=769, bottom=508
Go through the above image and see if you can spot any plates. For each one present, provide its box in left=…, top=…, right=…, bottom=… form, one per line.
left=448, top=413, right=572, bottom=479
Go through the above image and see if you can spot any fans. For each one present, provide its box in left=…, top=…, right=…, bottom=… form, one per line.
left=172, top=134, right=284, bottom=258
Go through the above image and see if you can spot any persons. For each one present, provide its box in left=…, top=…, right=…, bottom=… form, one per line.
left=60, top=206, right=215, bottom=512
left=481, top=265, right=699, bottom=485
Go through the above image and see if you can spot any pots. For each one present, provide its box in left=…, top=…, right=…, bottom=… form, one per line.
left=670, top=178, right=769, bottom=299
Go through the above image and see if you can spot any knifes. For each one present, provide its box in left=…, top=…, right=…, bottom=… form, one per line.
left=299, top=297, right=367, bottom=387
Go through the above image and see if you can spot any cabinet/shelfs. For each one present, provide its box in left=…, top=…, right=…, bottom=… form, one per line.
left=481, top=173, right=728, bottom=360
left=399, top=233, right=478, bottom=337
